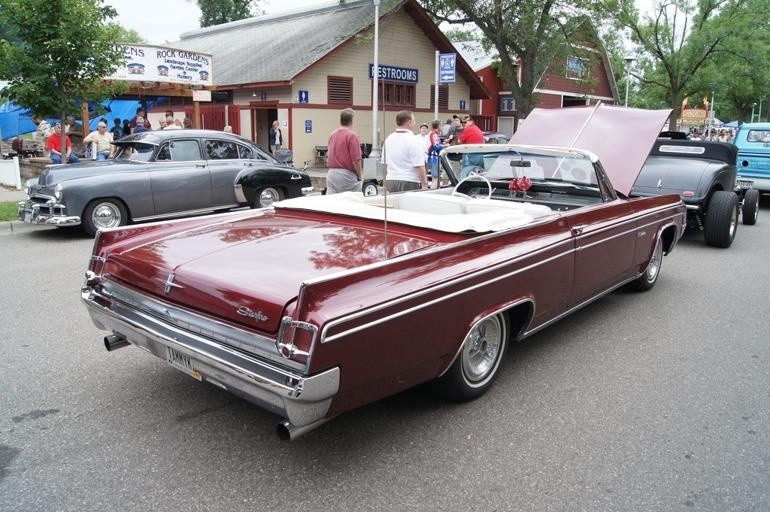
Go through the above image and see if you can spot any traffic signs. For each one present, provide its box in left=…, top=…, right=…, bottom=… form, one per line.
left=439, top=53, right=456, bottom=83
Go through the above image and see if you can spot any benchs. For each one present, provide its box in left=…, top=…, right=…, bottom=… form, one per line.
left=293, top=196, right=495, bottom=235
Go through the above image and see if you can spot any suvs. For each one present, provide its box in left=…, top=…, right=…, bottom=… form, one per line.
left=629, top=131, right=759, bottom=248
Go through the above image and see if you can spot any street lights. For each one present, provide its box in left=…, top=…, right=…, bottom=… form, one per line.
left=706, top=89, right=715, bottom=138
left=622, top=54, right=636, bottom=107
left=751, top=101, right=758, bottom=122
left=364, top=0, right=383, bottom=179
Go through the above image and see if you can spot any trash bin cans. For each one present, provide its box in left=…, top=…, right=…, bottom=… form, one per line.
left=274, top=150, right=292, bottom=167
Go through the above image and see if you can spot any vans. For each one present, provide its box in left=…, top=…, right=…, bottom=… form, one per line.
left=730, top=122, right=770, bottom=196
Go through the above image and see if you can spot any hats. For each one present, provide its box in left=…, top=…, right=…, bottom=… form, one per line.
left=453, top=114, right=471, bottom=123
left=419, top=123, right=428, bottom=127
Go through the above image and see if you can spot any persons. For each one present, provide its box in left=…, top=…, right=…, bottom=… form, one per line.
left=381, top=110, right=428, bottom=193
left=686, top=126, right=739, bottom=144
left=327, top=109, right=363, bottom=196
left=415, top=114, right=485, bottom=189
left=32, top=107, right=193, bottom=164
left=224, top=125, right=233, bottom=133
left=269, top=121, right=282, bottom=157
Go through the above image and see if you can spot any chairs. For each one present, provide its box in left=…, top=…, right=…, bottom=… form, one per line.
left=398, top=193, right=465, bottom=214
left=466, top=200, right=551, bottom=221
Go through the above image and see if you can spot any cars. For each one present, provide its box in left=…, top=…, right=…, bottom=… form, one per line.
left=463, top=129, right=509, bottom=176
left=18, top=128, right=312, bottom=237
left=82, top=98, right=689, bottom=443
